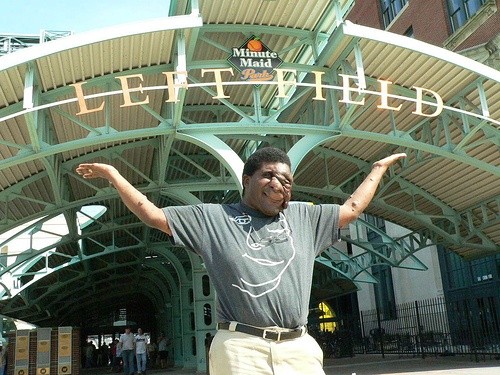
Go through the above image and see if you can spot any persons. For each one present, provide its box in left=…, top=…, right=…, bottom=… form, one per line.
left=76, top=147, right=407, bottom=375
left=134, top=328, right=149, bottom=375
left=83, top=330, right=172, bottom=370
left=119, top=328, right=135, bottom=375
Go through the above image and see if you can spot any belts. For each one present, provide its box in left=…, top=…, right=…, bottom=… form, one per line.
left=218, top=322, right=308, bottom=340
